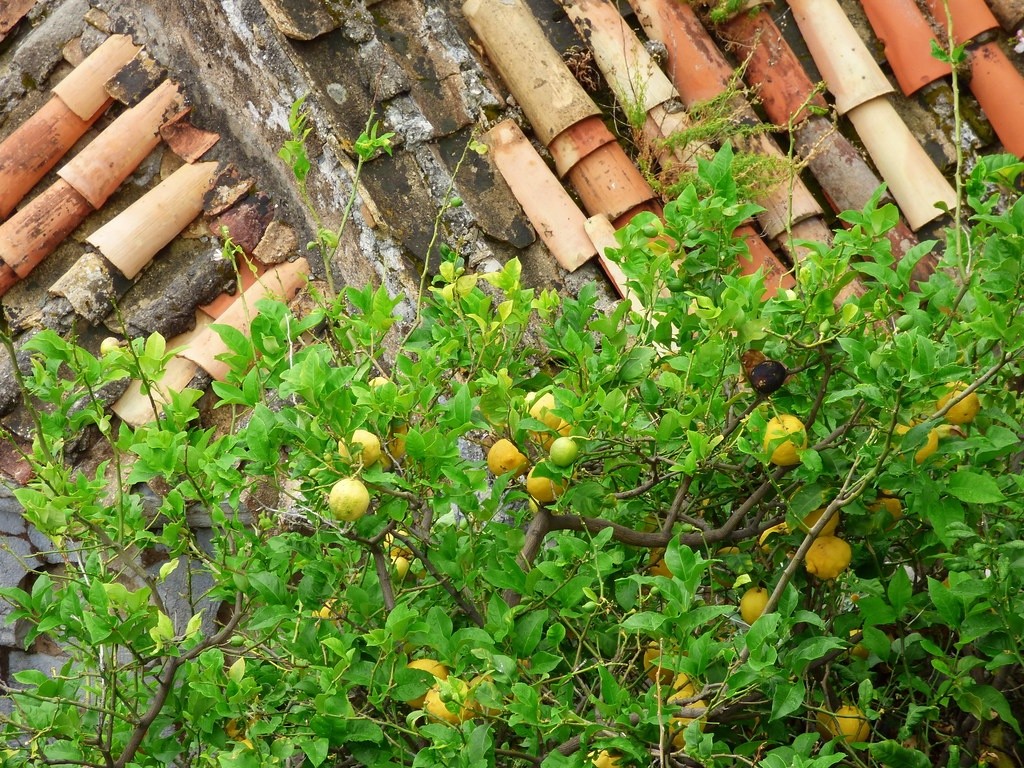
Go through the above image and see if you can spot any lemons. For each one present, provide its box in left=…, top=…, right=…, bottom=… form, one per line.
left=319, top=376, right=979, bottom=768
